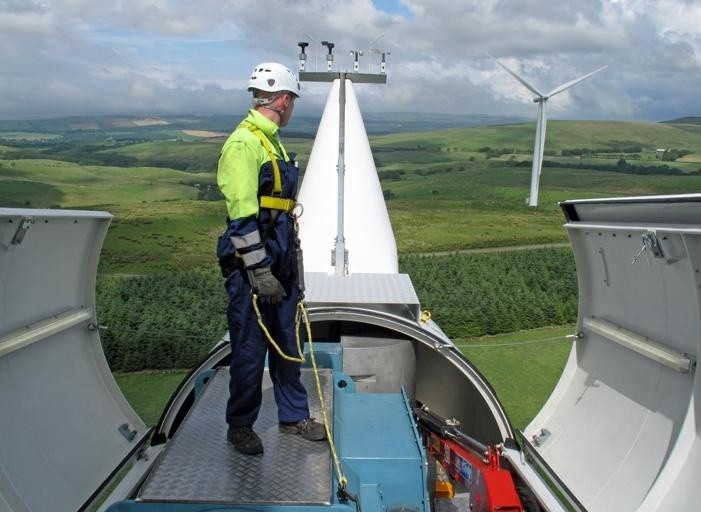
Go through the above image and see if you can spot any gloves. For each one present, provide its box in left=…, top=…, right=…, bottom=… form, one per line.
left=253, top=264, right=287, bottom=303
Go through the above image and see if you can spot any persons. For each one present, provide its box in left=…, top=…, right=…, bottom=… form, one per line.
left=214, top=61, right=330, bottom=455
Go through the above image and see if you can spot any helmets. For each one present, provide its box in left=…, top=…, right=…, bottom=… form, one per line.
left=248, top=61, right=302, bottom=99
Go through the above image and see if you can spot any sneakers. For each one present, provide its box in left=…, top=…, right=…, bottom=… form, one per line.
left=279, top=417, right=327, bottom=442
left=227, top=423, right=264, bottom=455
